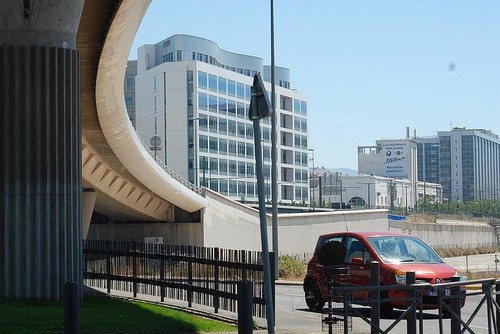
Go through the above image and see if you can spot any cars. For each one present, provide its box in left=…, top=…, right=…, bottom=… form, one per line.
left=303, top=230, right=467, bottom=319
